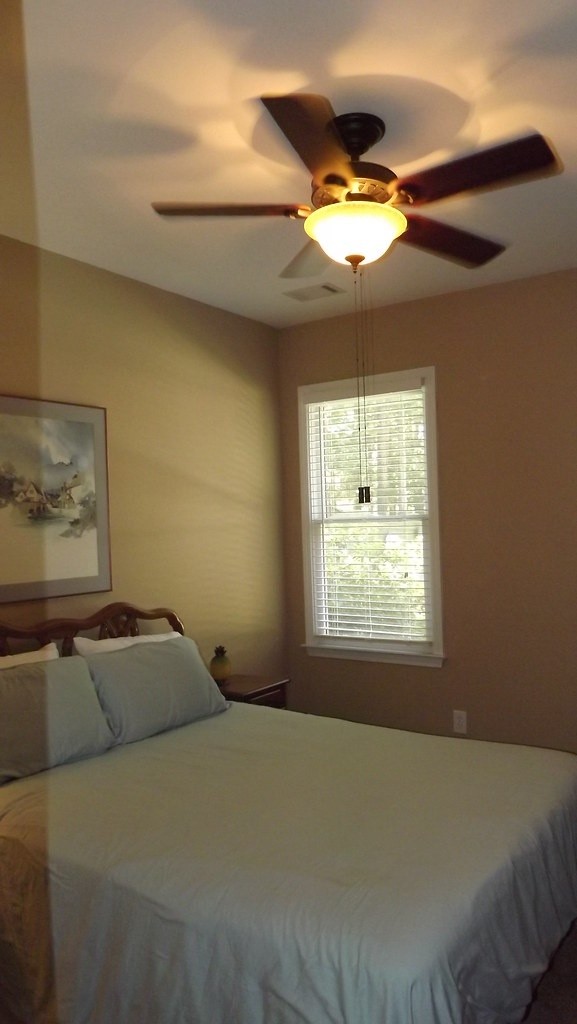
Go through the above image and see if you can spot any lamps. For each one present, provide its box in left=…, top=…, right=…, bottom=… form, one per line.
left=303, top=200, right=407, bottom=273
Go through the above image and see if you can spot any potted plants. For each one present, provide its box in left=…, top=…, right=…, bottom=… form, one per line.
left=210, top=646, right=231, bottom=687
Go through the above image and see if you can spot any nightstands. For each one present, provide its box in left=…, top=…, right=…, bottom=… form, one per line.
left=219, top=674, right=291, bottom=709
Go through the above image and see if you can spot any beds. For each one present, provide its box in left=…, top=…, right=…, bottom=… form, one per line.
left=2, top=603, right=577, bottom=1024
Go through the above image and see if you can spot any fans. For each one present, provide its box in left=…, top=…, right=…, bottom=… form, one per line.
left=150, top=94, right=564, bottom=281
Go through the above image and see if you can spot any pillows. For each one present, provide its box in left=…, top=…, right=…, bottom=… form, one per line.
left=73, top=631, right=181, bottom=653
left=0, top=654, right=118, bottom=784
left=0, top=642, right=60, bottom=669
left=85, top=636, right=231, bottom=744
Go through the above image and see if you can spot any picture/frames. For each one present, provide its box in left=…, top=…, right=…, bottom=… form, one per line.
left=0, top=395, right=112, bottom=604
